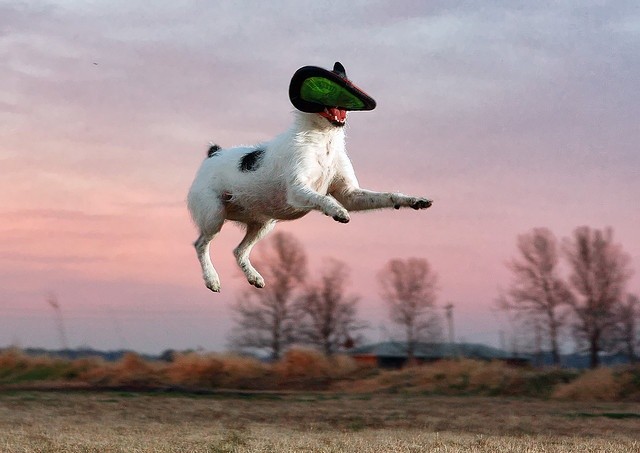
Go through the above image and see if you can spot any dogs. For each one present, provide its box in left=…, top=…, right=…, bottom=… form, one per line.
left=186, top=62, right=433, bottom=293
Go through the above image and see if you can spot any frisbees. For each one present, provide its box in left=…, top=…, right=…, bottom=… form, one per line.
left=290, top=66, right=376, bottom=113
